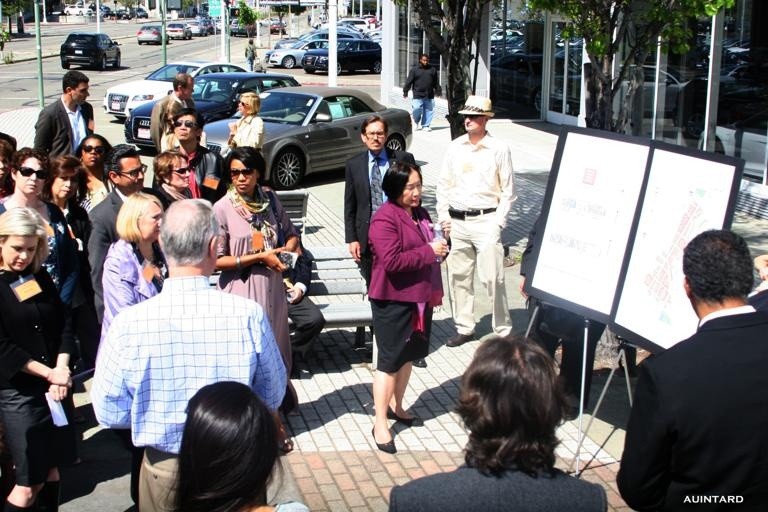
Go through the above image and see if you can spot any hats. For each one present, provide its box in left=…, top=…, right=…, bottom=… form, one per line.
left=458, top=95, right=495, bottom=117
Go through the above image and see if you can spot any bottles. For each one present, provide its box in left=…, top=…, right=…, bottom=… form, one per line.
left=432, top=222, right=446, bottom=263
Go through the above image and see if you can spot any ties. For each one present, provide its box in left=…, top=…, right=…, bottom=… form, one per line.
left=371, top=159, right=383, bottom=212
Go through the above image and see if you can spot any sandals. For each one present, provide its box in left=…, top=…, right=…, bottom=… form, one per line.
left=278, top=437, right=293, bottom=451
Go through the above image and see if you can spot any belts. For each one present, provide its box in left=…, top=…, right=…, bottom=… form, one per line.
left=448, top=208, right=496, bottom=215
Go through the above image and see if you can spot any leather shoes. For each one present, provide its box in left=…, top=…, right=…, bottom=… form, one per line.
left=372, top=407, right=422, bottom=453
left=412, top=357, right=427, bottom=367
left=447, top=334, right=472, bottom=346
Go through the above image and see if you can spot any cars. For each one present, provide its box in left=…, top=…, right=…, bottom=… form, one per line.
left=187, top=18, right=257, bottom=37
left=166, top=23, right=192, bottom=40
left=258, top=14, right=381, bottom=75
left=137, top=23, right=170, bottom=45
left=202, top=85, right=413, bottom=191
left=60, top=1, right=149, bottom=20
left=103, top=60, right=257, bottom=121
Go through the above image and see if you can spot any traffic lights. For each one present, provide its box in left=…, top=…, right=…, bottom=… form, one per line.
left=231, top=8, right=239, bottom=16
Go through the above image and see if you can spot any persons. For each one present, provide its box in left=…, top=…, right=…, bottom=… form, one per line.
left=388, top=333, right=610, bottom=512
left=364, top=157, right=453, bottom=455
left=518, top=216, right=607, bottom=422
left=243, top=39, right=259, bottom=71
left=442, top=92, right=520, bottom=348
left=616, top=229, right=768, bottom=512
left=402, top=53, right=443, bottom=132
left=343, top=113, right=428, bottom=368
left=746, top=254, right=768, bottom=311
left=307, top=13, right=312, bottom=25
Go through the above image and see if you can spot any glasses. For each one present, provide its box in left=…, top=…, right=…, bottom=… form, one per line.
left=231, top=169, right=251, bottom=175
left=175, top=120, right=194, bottom=126
left=16, top=167, right=47, bottom=179
left=83, top=145, right=104, bottom=153
left=172, top=167, right=191, bottom=173
left=121, top=165, right=147, bottom=176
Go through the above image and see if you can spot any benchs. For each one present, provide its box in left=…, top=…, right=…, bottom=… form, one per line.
left=274, top=192, right=309, bottom=244
left=161, top=244, right=379, bottom=373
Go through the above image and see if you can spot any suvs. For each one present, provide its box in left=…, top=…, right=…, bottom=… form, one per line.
left=122, top=72, right=304, bottom=153
left=61, top=31, right=121, bottom=70
